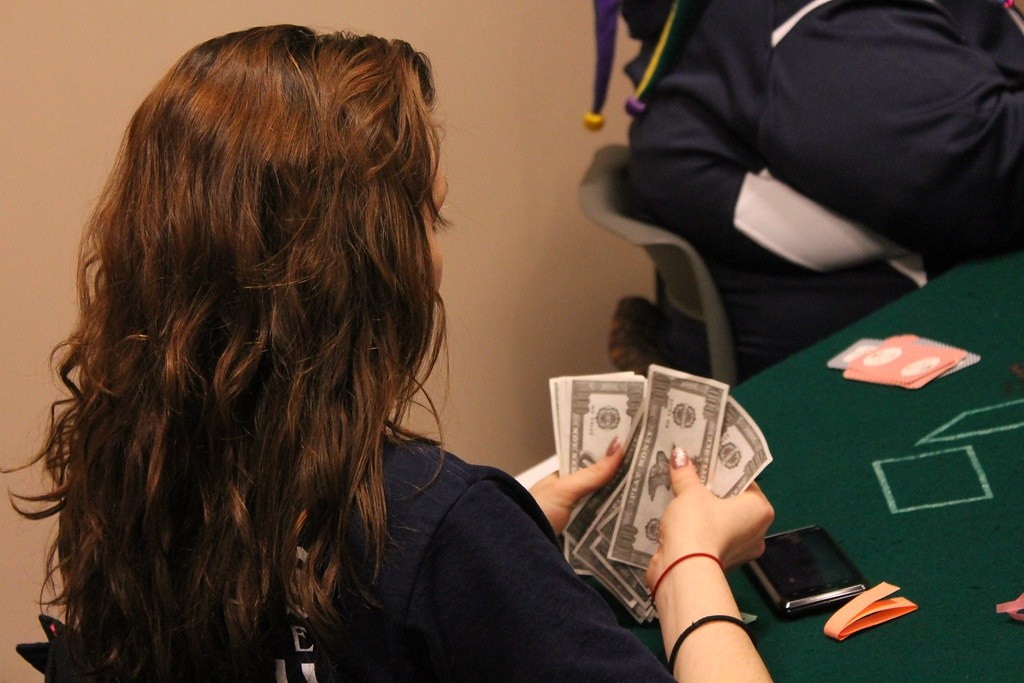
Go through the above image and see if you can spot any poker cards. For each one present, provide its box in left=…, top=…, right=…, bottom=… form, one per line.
left=826, top=331, right=983, bottom=392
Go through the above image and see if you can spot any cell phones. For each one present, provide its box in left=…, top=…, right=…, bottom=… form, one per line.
left=748, top=524, right=869, bottom=621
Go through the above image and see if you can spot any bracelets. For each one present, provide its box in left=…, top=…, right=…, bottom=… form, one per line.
left=651, top=553, right=724, bottom=607
left=669, top=615, right=758, bottom=673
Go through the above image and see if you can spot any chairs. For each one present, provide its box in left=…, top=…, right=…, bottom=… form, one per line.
left=579, top=142, right=740, bottom=396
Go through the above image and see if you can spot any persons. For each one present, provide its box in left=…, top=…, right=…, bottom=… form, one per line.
left=588, top=0, right=1024, bottom=385
left=15, top=24, right=775, bottom=682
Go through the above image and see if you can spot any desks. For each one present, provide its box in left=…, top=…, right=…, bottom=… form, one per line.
left=572, top=245, right=1024, bottom=683
